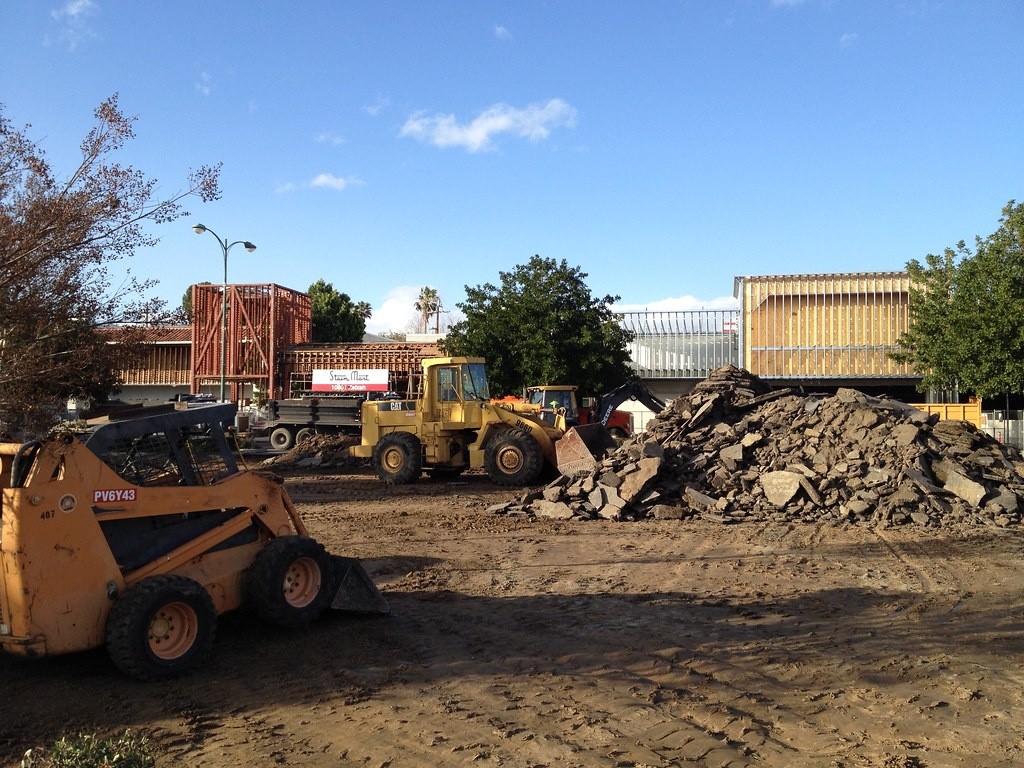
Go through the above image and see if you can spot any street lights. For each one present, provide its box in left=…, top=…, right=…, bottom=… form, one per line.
left=191, top=222, right=258, bottom=406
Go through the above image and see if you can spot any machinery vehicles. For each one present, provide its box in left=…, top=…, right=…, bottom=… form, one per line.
left=0, top=397, right=390, bottom=694
left=523, top=374, right=667, bottom=450
left=350, top=356, right=625, bottom=490
